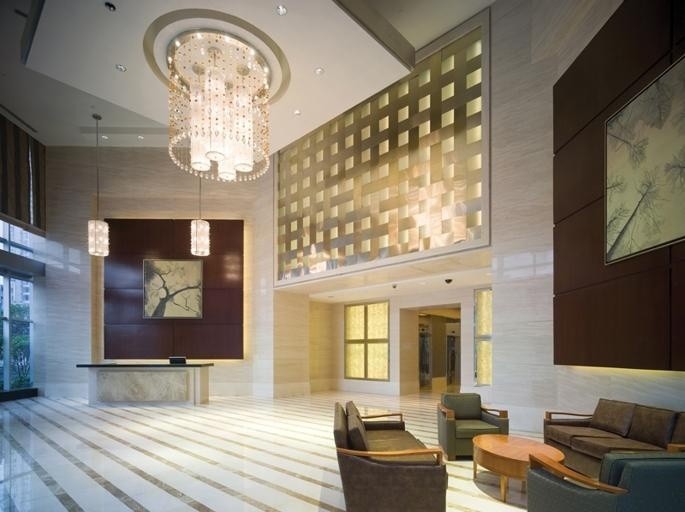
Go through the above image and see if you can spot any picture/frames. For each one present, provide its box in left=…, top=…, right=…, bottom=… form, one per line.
left=142, top=258, right=204, bottom=319
left=603, top=54, right=685, bottom=267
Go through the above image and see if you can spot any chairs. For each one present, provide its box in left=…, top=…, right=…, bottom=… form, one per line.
left=527, top=450, right=685, bottom=512
left=438, top=393, right=509, bottom=461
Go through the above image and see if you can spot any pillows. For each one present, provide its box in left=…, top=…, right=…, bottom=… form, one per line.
left=628, top=405, right=678, bottom=450
left=599, top=452, right=685, bottom=487
left=441, top=393, right=482, bottom=420
left=590, top=398, right=637, bottom=437
left=348, top=415, right=368, bottom=450
left=672, top=412, right=685, bottom=444
left=346, top=401, right=361, bottom=418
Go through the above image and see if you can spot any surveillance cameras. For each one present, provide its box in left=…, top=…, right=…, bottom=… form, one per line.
left=392, top=283, right=397, bottom=289
left=445, top=279, right=453, bottom=284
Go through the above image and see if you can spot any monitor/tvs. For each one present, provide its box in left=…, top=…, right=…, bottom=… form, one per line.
left=170, top=356, right=186, bottom=363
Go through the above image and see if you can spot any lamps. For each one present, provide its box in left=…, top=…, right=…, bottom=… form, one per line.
left=190, top=177, right=210, bottom=256
left=166, top=29, right=270, bottom=183
left=88, top=113, right=110, bottom=257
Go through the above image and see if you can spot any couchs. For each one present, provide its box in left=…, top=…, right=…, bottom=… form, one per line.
left=543, top=398, right=685, bottom=479
left=333, top=401, right=449, bottom=512
